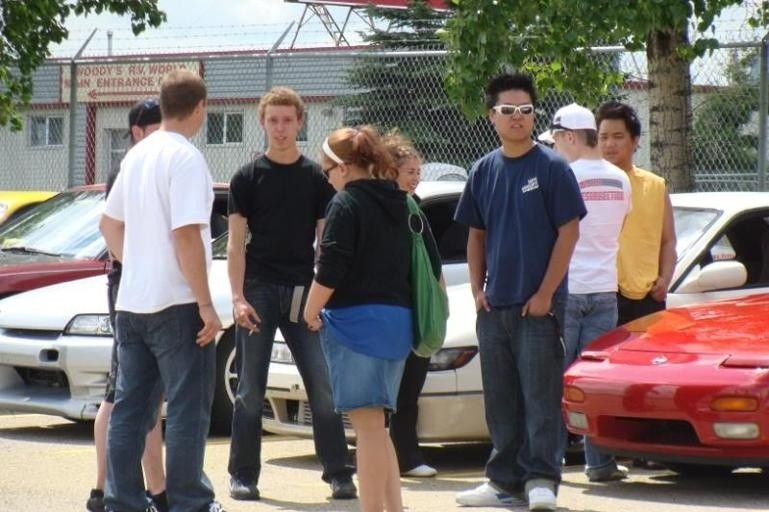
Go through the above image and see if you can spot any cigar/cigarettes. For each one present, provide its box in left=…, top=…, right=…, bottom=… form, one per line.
left=247, top=324, right=256, bottom=337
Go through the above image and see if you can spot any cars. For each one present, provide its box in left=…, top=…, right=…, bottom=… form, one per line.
left=562, top=293, right=769, bottom=484
left=261, top=190, right=769, bottom=463
left=0, top=174, right=466, bottom=447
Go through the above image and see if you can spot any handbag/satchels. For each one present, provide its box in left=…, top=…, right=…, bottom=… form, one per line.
left=403, top=193, right=450, bottom=359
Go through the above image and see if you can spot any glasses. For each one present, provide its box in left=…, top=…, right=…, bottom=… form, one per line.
left=491, top=102, right=534, bottom=117
left=135, top=100, right=160, bottom=126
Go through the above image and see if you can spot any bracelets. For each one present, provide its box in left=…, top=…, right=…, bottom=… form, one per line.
left=198, top=301, right=213, bottom=308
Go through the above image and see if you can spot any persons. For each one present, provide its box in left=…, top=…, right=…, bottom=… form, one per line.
left=99, top=67, right=230, bottom=512
left=85, top=100, right=170, bottom=512
left=227, top=88, right=357, bottom=498
left=597, top=101, right=676, bottom=470
left=380, top=128, right=437, bottom=477
left=303, top=125, right=447, bottom=512
left=453, top=75, right=587, bottom=512
left=537, top=102, right=632, bottom=481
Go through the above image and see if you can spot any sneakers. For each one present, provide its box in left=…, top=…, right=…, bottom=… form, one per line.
left=331, top=475, right=357, bottom=499
left=400, top=463, right=438, bottom=477
left=230, top=474, right=259, bottom=500
left=589, top=463, right=629, bottom=481
left=87, top=489, right=223, bottom=512
left=455, top=481, right=528, bottom=507
left=525, top=479, right=558, bottom=512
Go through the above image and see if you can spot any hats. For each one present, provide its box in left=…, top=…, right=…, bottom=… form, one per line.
left=120, top=103, right=158, bottom=139
left=536, top=103, right=598, bottom=142
